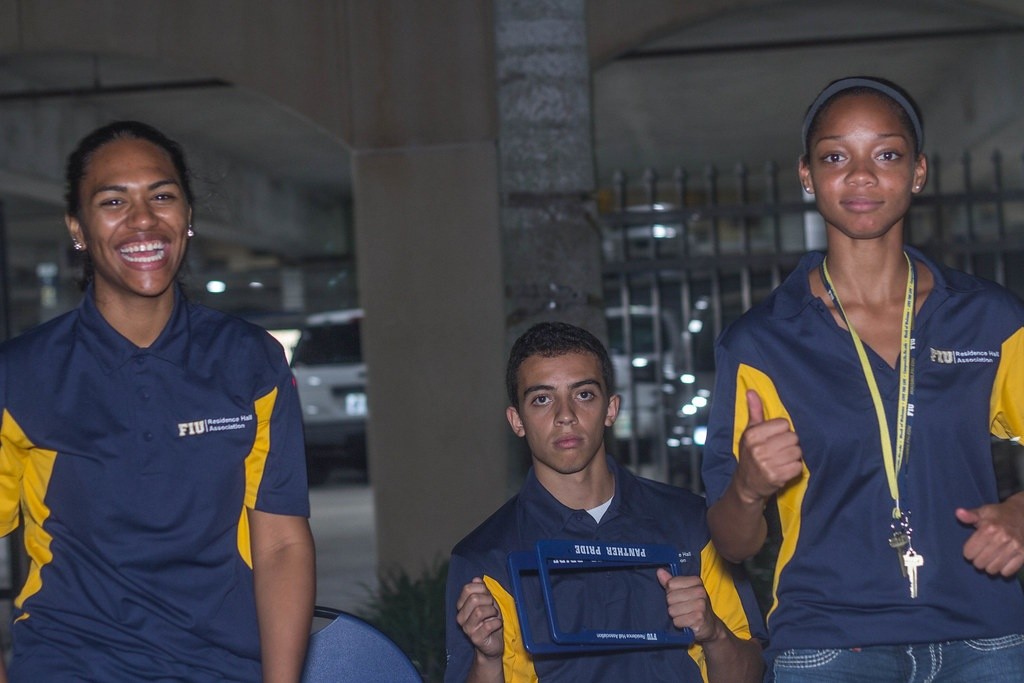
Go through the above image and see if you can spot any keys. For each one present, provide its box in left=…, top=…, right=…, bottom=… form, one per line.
left=888, top=532, right=909, bottom=577
left=903, top=548, right=924, bottom=599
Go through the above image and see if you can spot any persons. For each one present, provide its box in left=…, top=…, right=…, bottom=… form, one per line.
left=0, top=120, right=313, bottom=683
left=443, top=324, right=768, bottom=683
left=700, top=78, right=1024, bottom=683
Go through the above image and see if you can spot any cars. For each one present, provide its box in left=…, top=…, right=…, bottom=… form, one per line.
left=604, top=303, right=689, bottom=442
left=287, top=307, right=365, bottom=472
